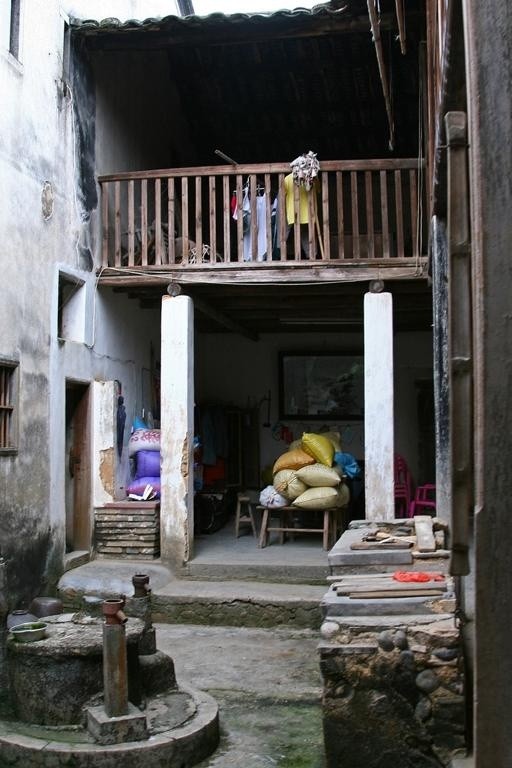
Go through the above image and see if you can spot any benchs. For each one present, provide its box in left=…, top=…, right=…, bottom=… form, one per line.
left=258, top=505, right=350, bottom=552
left=235, top=492, right=257, bottom=537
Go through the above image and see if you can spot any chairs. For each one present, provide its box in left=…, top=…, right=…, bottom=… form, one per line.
left=392, top=452, right=436, bottom=518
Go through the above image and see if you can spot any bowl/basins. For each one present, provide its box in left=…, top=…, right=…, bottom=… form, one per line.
left=8, top=621, right=49, bottom=642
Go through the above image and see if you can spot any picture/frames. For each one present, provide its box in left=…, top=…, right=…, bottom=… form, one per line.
left=276, top=349, right=365, bottom=421
